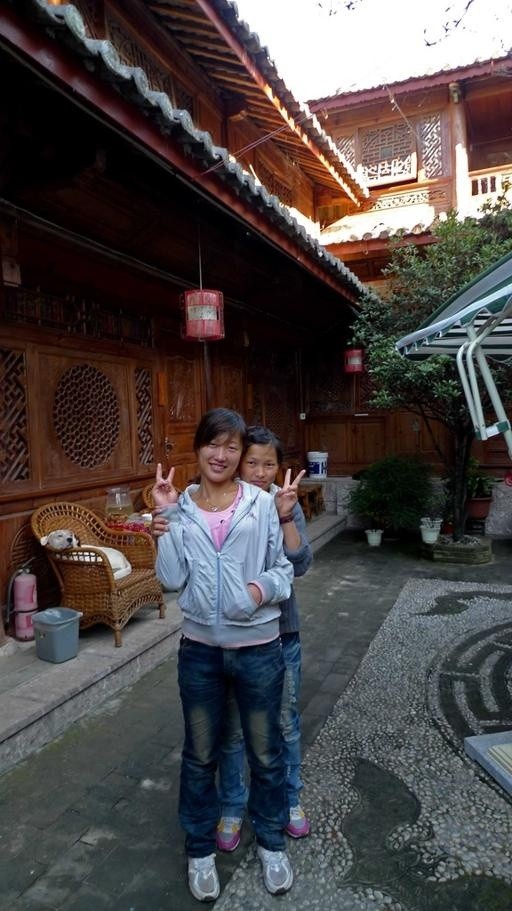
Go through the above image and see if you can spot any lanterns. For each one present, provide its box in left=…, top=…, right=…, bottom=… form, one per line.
left=179, top=289, right=225, bottom=341
left=343, top=348, right=367, bottom=376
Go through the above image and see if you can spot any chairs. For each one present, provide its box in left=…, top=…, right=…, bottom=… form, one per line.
left=275, top=458, right=323, bottom=521
left=143, top=482, right=183, bottom=512
left=31, top=501, right=165, bottom=648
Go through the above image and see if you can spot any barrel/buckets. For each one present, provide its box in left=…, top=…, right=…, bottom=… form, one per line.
left=304, top=450, right=330, bottom=480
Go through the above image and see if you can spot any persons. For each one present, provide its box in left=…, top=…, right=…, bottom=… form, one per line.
left=151, top=426, right=312, bottom=851
left=151, top=407, right=295, bottom=901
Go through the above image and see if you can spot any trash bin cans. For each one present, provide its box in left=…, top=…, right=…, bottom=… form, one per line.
left=32, top=607, right=83, bottom=663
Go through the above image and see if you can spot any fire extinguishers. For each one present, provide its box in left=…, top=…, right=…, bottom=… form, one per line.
left=5, top=557, right=39, bottom=641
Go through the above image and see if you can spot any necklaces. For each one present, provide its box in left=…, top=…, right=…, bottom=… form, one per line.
left=197, top=485, right=237, bottom=512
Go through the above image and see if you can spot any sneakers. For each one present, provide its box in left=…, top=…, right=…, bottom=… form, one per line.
left=256, top=843, right=293, bottom=894
left=285, top=804, right=310, bottom=838
left=219, top=816, right=244, bottom=851
left=186, top=850, right=220, bottom=902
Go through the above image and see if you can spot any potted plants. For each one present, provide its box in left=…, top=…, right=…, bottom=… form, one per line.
left=467, top=470, right=497, bottom=520
left=347, top=453, right=453, bottom=547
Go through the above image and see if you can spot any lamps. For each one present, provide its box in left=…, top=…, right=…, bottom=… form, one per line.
left=346, top=309, right=363, bottom=374
left=185, top=217, right=224, bottom=343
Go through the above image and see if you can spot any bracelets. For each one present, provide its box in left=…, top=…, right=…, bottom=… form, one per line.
left=280, top=513, right=294, bottom=524
